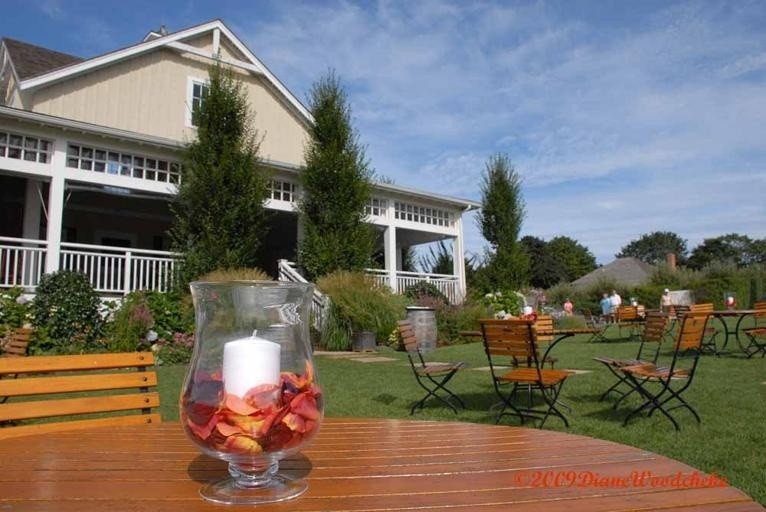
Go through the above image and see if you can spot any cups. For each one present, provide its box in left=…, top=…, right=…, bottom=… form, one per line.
left=517, top=297, right=537, bottom=320
left=179, top=277, right=323, bottom=506
left=630, top=296, right=638, bottom=307
left=723, top=292, right=737, bottom=311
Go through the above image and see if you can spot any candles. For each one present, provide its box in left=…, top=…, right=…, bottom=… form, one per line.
left=219, top=335, right=281, bottom=399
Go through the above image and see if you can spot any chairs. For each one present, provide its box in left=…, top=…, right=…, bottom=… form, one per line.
left=396, top=302, right=765, bottom=431
left=0, top=327, right=162, bottom=439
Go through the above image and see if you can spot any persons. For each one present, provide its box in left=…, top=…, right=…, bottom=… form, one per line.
left=484, top=287, right=674, bottom=328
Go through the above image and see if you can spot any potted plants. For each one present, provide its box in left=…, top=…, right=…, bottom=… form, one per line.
left=347, top=303, right=377, bottom=354
